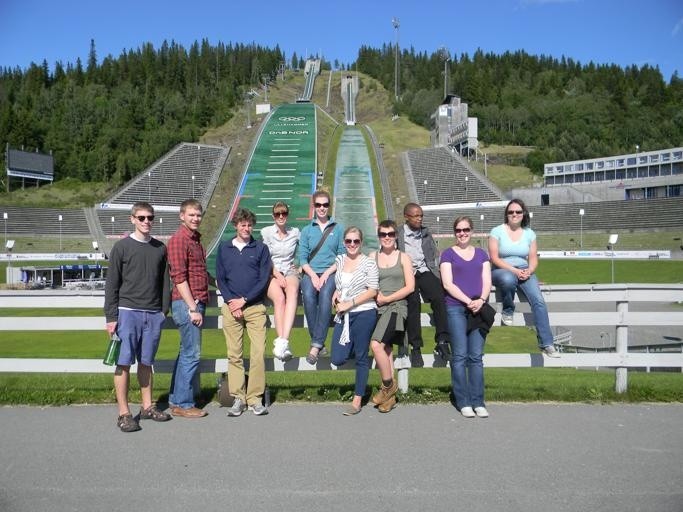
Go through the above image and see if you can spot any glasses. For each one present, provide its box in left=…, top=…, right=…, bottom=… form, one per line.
left=507, top=211, right=523, bottom=214
left=274, top=212, right=288, bottom=218
left=134, top=216, right=154, bottom=222
left=345, top=239, right=360, bottom=244
left=314, top=203, right=329, bottom=208
left=455, top=228, right=470, bottom=233
left=379, top=232, right=395, bottom=238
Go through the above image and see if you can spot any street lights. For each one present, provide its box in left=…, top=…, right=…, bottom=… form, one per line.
left=58, top=214, right=62, bottom=251
left=195, top=144, right=201, bottom=166
left=3, top=211, right=8, bottom=251
left=424, top=173, right=618, bottom=284
left=110, top=216, right=115, bottom=235
left=437, top=47, right=451, bottom=102
left=92, top=241, right=98, bottom=265
left=390, top=14, right=401, bottom=98
left=190, top=175, right=195, bottom=198
left=4, top=239, right=15, bottom=288
left=158, top=217, right=163, bottom=237
left=147, top=172, right=151, bottom=201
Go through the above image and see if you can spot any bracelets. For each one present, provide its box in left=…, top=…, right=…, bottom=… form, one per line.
left=350, top=297, right=356, bottom=308
left=478, top=298, right=488, bottom=304
left=188, top=305, right=200, bottom=313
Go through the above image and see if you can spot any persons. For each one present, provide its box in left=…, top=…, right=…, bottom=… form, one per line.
left=104, top=202, right=171, bottom=431
left=394, top=202, right=451, bottom=368
left=298, top=190, right=347, bottom=365
left=330, top=225, right=379, bottom=416
left=365, top=220, right=415, bottom=413
left=439, top=216, right=491, bottom=419
left=166, top=199, right=218, bottom=419
left=485, top=197, right=562, bottom=359
left=256, top=200, right=303, bottom=362
left=213, top=206, right=274, bottom=416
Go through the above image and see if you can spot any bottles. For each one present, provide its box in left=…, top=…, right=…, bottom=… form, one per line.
left=101, top=331, right=121, bottom=366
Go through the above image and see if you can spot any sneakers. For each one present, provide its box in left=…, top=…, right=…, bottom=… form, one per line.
left=474, top=406, right=489, bottom=416
left=172, top=406, right=207, bottom=417
left=118, top=415, right=140, bottom=432
left=248, top=404, right=268, bottom=414
left=540, top=345, right=560, bottom=358
left=373, top=379, right=398, bottom=404
left=461, top=407, right=475, bottom=417
left=140, top=403, right=170, bottom=421
left=228, top=398, right=247, bottom=416
left=378, top=395, right=396, bottom=412
left=502, top=313, right=513, bottom=326
left=306, top=347, right=327, bottom=364
left=343, top=405, right=361, bottom=415
left=434, top=343, right=453, bottom=361
left=411, top=347, right=424, bottom=367
left=273, top=337, right=294, bottom=365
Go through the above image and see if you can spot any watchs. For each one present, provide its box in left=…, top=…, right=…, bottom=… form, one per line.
left=243, top=295, right=248, bottom=302
